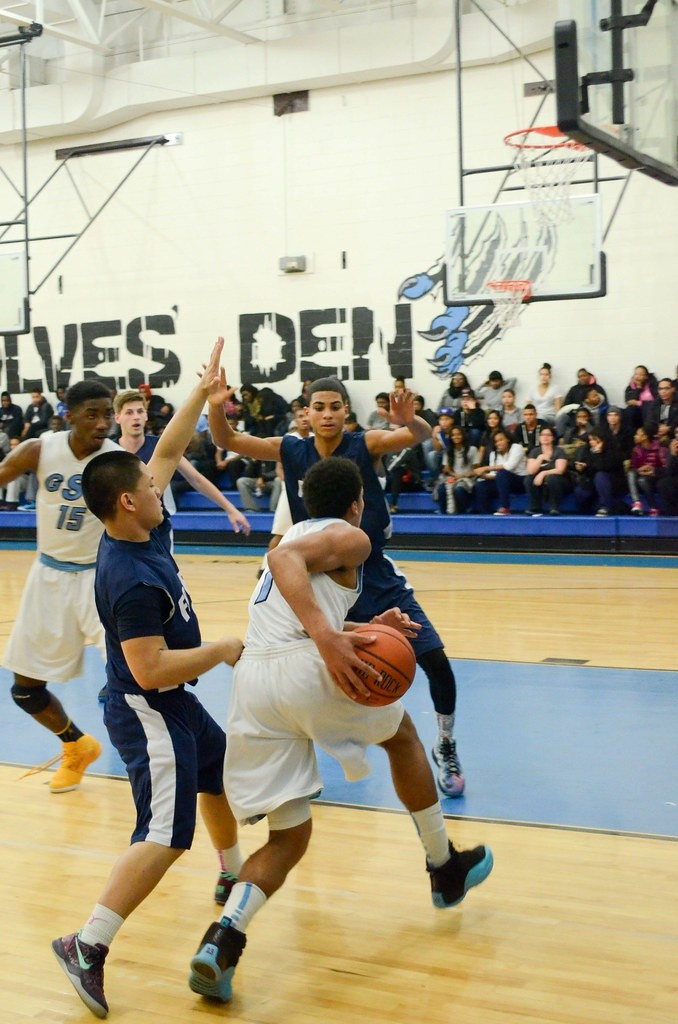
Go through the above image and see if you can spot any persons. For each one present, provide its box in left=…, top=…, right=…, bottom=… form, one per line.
left=54, top=336, right=243, bottom=1018
left=344, top=363, right=678, bottom=517
left=0, top=380, right=125, bottom=793
left=139, top=379, right=314, bottom=513
left=0, top=386, right=72, bottom=511
left=114, top=391, right=252, bottom=554
left=189, top=457, right=493, bottom=1002
left=198, top=364, right=465, bottom=797
left=257, top=410, right=315, bottom=575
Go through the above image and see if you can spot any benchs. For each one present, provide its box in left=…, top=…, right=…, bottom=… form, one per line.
left=0, top=472, right=678, bottom=556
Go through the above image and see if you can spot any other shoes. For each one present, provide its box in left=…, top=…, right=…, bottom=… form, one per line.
left=389, top=503, right=399, bottom=514
left=549, top=507, right=560, bottom=515
left=596, top=509, right=609, bottom=516
left=649, top=508, right=659, bottom=517
left=493, top=507, right=511, bottom=516
left=631, top=500, right=644, bottom=515
left=0, top=502, right=16, bottom=511
left=17, top=500, right=35, bottom=512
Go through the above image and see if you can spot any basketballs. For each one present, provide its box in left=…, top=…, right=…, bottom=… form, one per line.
left=335, top=624, right=417, bottom=708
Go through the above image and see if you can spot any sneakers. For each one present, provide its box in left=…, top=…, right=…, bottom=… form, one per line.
left=432, top=736, right=465, bottom=797
left=215, top=870, right=238, bottom=904
left=426, top=839, right=493, bottom=908
left=189, top=916, right=246, bottom=1004
left=52, top=931, right=109, bottom=1019
left=20, top=734, right=102, bottom=792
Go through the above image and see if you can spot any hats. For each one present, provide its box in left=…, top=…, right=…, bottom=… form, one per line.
left=460, top=388, right=473, bottom=398
left=438, top=407, right=454, bottom=418
